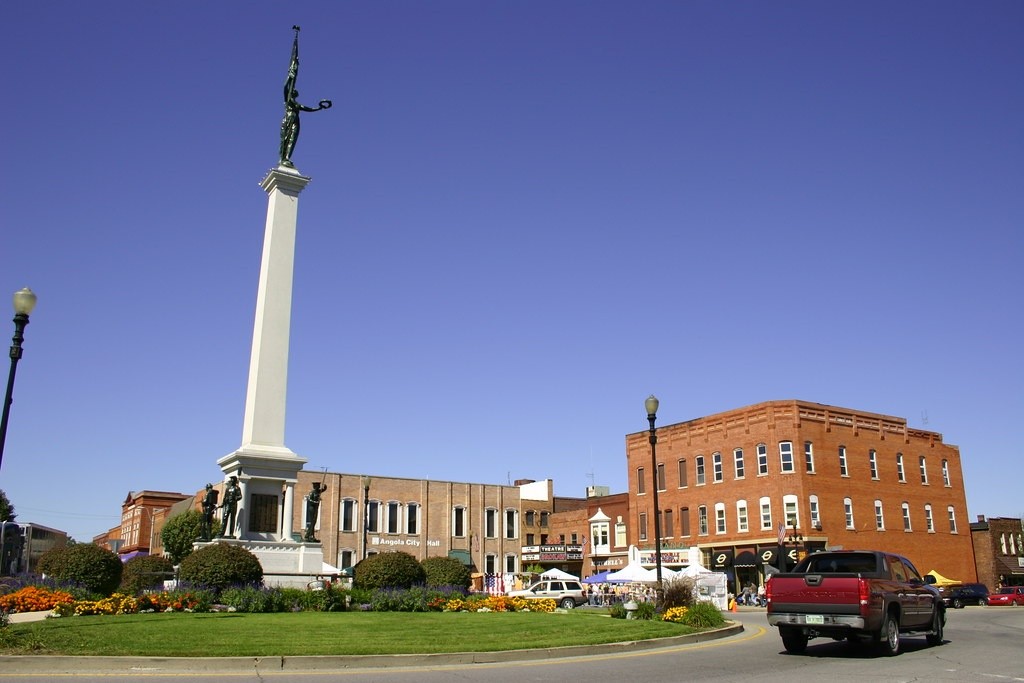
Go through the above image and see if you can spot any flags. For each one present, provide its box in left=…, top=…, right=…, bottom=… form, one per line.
left=778, top=523, right=786, bottom=545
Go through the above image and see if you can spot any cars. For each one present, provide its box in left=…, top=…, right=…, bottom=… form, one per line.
left=986, top=585, right=1024, bottom=608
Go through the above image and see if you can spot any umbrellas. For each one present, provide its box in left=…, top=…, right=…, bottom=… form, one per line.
left=538, top=563, right=712, bottom=604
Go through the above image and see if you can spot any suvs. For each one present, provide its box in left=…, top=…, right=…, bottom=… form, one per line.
left=508, top=579, right=589, bottom=610
left=940, top=582, right=990, bottom=609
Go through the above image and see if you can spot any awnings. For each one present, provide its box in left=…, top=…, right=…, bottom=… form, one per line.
left=997, top=556, right=1024, bottom=574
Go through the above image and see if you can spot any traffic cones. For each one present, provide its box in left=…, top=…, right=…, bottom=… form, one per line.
left=732, top=603, right=738, bottom=613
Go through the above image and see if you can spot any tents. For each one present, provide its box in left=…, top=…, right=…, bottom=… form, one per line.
left=923, top=570, right=961, bottom=587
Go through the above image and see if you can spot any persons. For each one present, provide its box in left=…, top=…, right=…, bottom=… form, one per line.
left=201, top=483, right=215, bottom=539
left=741, top=581, right=764, bottom=607
left=304, top=482, right=327, bottom=543
left=214, top=476, right=243, bottom=537
left=280, top=75, right=320, bottom=164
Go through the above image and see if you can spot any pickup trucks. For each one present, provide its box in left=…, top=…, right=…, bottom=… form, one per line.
left=765, top=549, right=949, bottom=657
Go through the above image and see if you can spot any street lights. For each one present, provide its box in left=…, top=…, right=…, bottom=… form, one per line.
left=362, top=474, right=371, bottom=561
left=468, top=529, right=473, bottom=572
left=593, top=530, right=598, bottom=574
left=0, top=286, right=38, bottom=467
left=644, top=394, right=667, bottom=607
left=137, top=505, right=155, bottom=556
left=788, top=516, right=804, bottom=563
